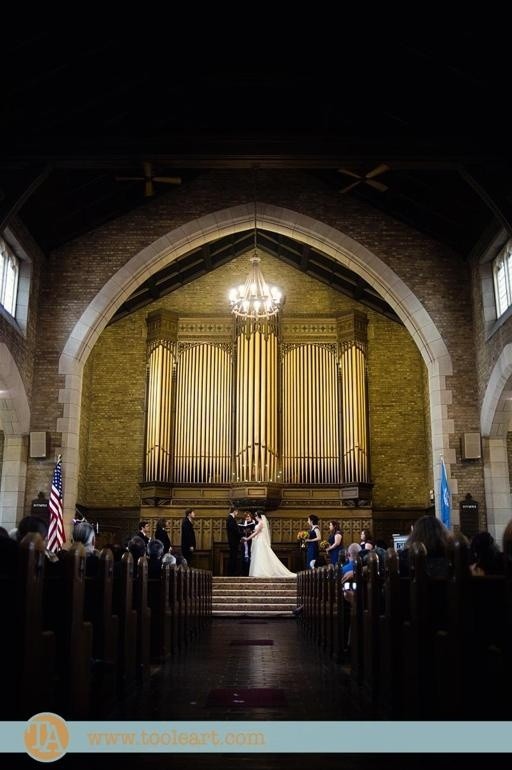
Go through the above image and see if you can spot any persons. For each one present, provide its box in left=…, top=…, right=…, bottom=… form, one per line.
left=1, top=514, right=512, bottom=607
left=180, top=508, right=196, bottom=566
left=237, top=511, right=255, bottom=525
left=225, top=506, right=246, bottom=576
left=241, top=511, right=297, bottom=577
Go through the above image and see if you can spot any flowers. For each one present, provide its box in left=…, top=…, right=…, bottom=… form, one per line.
left=319, top=541, right=330, bottom=551
left=296, top=531, right=310, bottom=548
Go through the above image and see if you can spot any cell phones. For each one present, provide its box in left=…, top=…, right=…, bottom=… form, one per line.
left=342, top=579, right=350, bottom=591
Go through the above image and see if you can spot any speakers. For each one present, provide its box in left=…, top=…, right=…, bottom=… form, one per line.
left=31, top=499, right=50, bottom=524
left=464, top=432, right=481, bottom=458
left=460, top=500, right=479, bottom=541
left=30, top=431, right=46, bottom=457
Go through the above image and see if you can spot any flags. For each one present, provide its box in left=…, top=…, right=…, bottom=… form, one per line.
left=46, top=458, right=67, bottom=553
left=437, top=460, right=452, bottom=530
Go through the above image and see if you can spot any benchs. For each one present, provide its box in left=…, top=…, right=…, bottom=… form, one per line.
left=0, top=536, right=213, bottom=720
left=296, top=552, right=512, bottom=721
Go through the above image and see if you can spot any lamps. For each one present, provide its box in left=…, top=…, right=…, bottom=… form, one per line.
left=228, top=202, right=284, bottom=321
